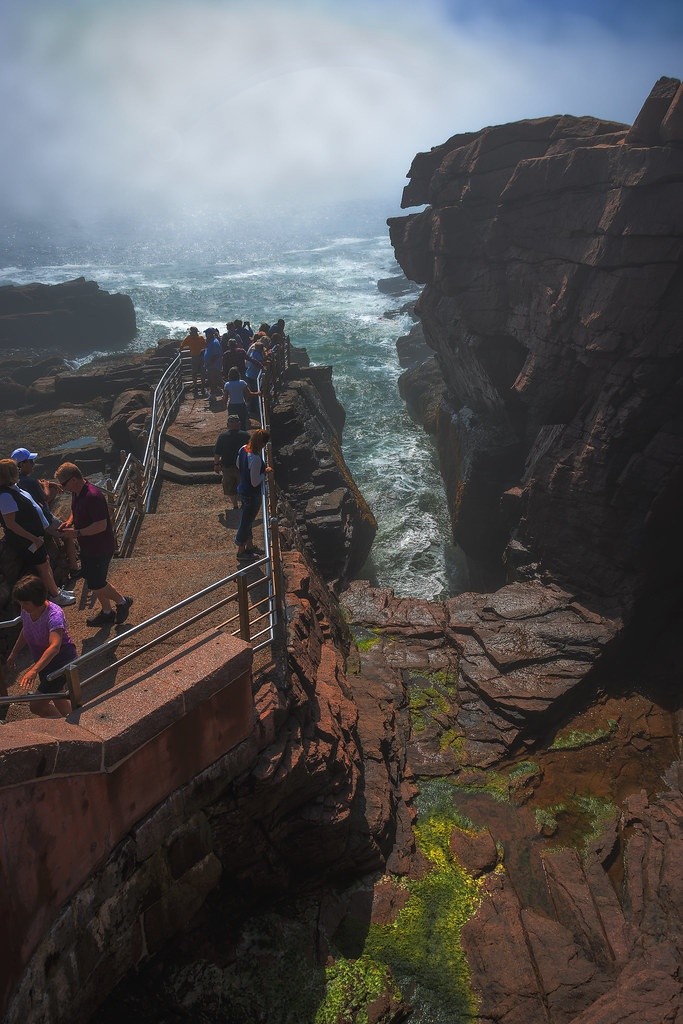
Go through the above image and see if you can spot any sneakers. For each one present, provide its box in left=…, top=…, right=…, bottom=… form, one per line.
left=86, top=610, right=115, bottom=626
left=48, top=589, right=76, bottom=605
left=58, top=587, right=75, bottom=596
left=114, top=596, right=133, bottom=624
left=245, top=545, right=265, bottom=557
left=236, top=549, right=259, bottom=560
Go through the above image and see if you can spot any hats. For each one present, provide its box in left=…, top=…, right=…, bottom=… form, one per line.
left=11, top=448, right=38, bottom=463
left=227, top=414, right=240, bottom=424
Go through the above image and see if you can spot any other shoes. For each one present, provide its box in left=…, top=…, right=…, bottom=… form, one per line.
left=70, top=568, right=82, bottom=580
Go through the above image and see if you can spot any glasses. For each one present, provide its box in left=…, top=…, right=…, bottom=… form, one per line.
left=61, top=477, right=72, bottom=487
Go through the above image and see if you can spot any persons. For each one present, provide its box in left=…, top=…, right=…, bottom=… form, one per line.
left=214, top=415, right=251, bottom=510
left=54, top=462, right=134, bottom=627
left=204, top=319, right=286, bottom=401
left=235, top=429, right=274, bottom=560
left=223, top=366, right=261, bottom=431
left=177, top=327, right=207, bottom=393
left=0, top=448, right=81, bottom=605
left=6, top=575, right=78, bottom=718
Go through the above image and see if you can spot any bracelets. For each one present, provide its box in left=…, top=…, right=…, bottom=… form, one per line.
left=215, top=464, right=218, bottom=466
left=76, top=529, right=80, bottom=538
